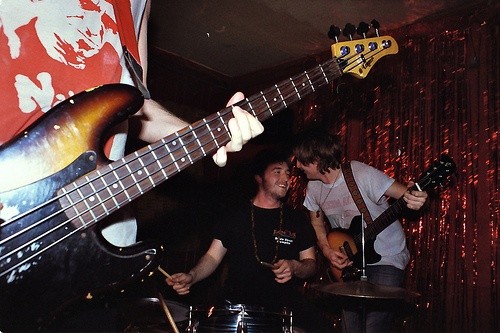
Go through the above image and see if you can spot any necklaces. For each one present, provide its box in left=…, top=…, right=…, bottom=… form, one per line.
left=250, top=200, right=284, bottom=265
left=316, top=168, right=342, bottom=218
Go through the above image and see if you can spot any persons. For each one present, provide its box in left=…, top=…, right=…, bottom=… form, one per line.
left=166, top=152, right=318, bottom=306
left=0, top=1, right=264, bottom=332
left=292, top=126, right=428, bottom=333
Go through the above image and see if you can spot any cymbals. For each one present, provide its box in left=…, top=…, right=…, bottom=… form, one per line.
left=114, top=294, right=193, bottom=326
left=308, top=279, right=413, bottom=300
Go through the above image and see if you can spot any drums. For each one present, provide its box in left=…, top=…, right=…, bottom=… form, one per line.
left=202, top=303, right=342, bottom=333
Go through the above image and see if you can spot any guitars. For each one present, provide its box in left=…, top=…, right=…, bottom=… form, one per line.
left=324, top=152, right=457, bottom=282
left=0, top=17, right=403, bottom=333
